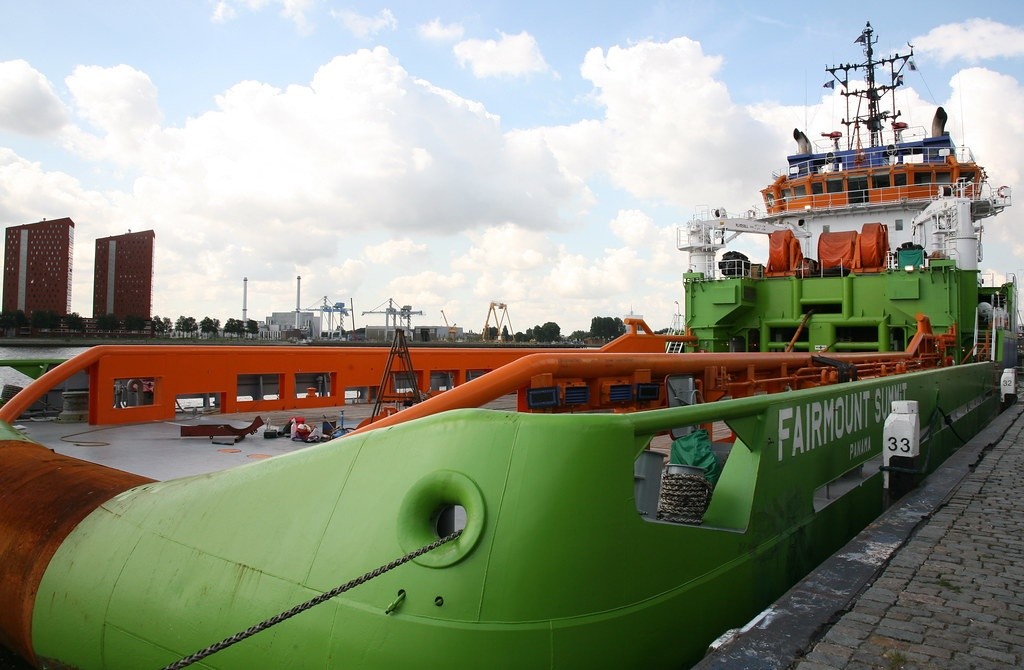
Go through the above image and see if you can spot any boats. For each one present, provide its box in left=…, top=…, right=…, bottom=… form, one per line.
left=0, top=19, right=1024, bottom=670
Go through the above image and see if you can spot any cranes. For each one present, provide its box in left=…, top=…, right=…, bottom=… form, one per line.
left=361, top=297, right=422, bottom=342
left=300, top=295, right=351, bottom=338
left=440, top=309, right=457, bottom=341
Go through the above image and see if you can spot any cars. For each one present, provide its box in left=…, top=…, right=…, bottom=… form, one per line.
left=321, top=335, right=371, bottom=342
left=562, top=341, right=585, bottom=344
